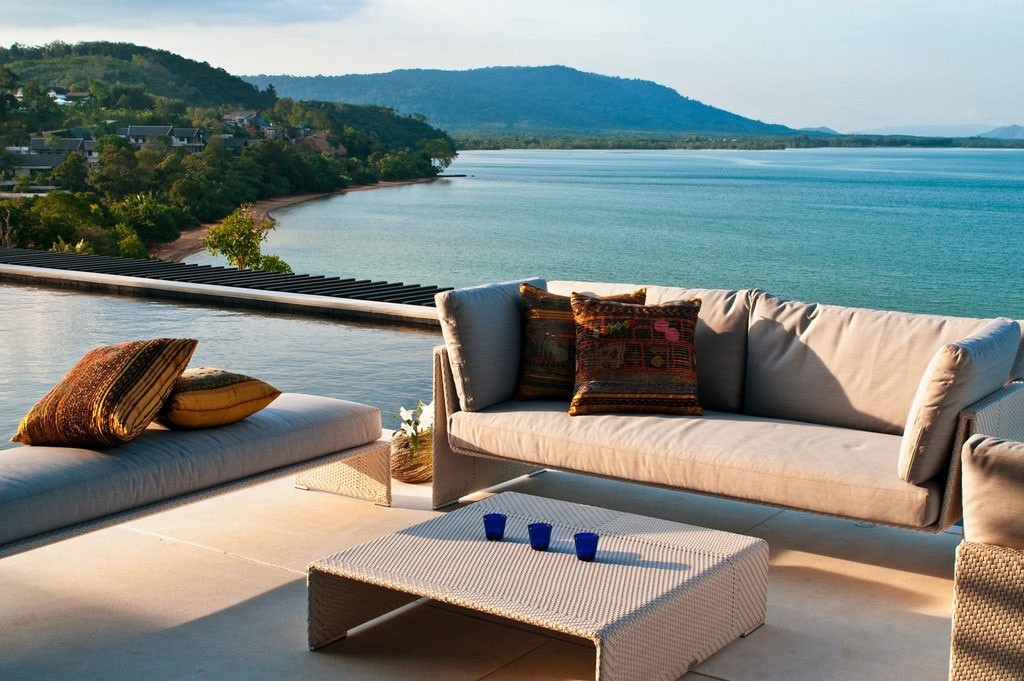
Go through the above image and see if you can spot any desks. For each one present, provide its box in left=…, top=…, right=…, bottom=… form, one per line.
left=306, top=490, right=771, bottom=681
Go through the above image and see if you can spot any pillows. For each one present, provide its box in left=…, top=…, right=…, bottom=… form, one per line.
left=9, top=338, right=198, bottom=448
left=570, top=291, right=705, bottom=417
left=897, top=316, right=1021, bottom=484
left=517, top=283, right=646, bottom=400
left=436, top=275, right=549, bottom=411
left=153, top=368, right=282, bottom=431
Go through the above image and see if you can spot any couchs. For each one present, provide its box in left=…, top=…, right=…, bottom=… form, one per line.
left=431, top=278, right=1024, bottom=531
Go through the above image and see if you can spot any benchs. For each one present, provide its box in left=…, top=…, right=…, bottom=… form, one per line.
left=0, top=393, right=391, bottom=550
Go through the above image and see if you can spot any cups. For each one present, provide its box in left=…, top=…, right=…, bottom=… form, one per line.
left=528, top=522, right=552, bottom=551
left=483, top=513, right=507, bottom=540
left=574, top=532, right=599, bottom=561
